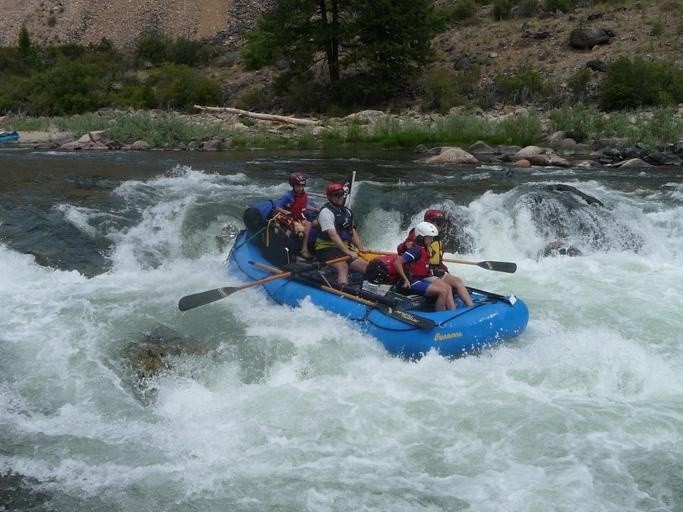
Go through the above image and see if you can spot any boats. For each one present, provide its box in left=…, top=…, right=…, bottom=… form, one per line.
left=226, top=198, right=529, bottom=364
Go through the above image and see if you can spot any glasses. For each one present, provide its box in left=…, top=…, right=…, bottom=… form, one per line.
left=334, top=192, right=344, bottom=197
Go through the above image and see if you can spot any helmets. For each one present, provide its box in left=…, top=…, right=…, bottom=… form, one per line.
left=414, top=221, right=439, bottom=237
left=289, top=172, right=306, bottom=185
left=425, top=210, right=445, bottom=221
left=327, top=182, right=344, bottom=195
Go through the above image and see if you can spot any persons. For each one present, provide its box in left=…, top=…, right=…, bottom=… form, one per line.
left=276, top=173, right=320, bottom=258
left=315, top=184, right=369, bottom=284
left=393, top=222, right=449, bottom=311
left=397, top=210, right=473, bottom=310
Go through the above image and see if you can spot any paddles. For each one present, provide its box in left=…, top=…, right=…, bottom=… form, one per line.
left=248, top=261, right=435, bottom=328
left=179, top=249, right=361, bottom=311
left=364, top=250, right=516, bottom=273
